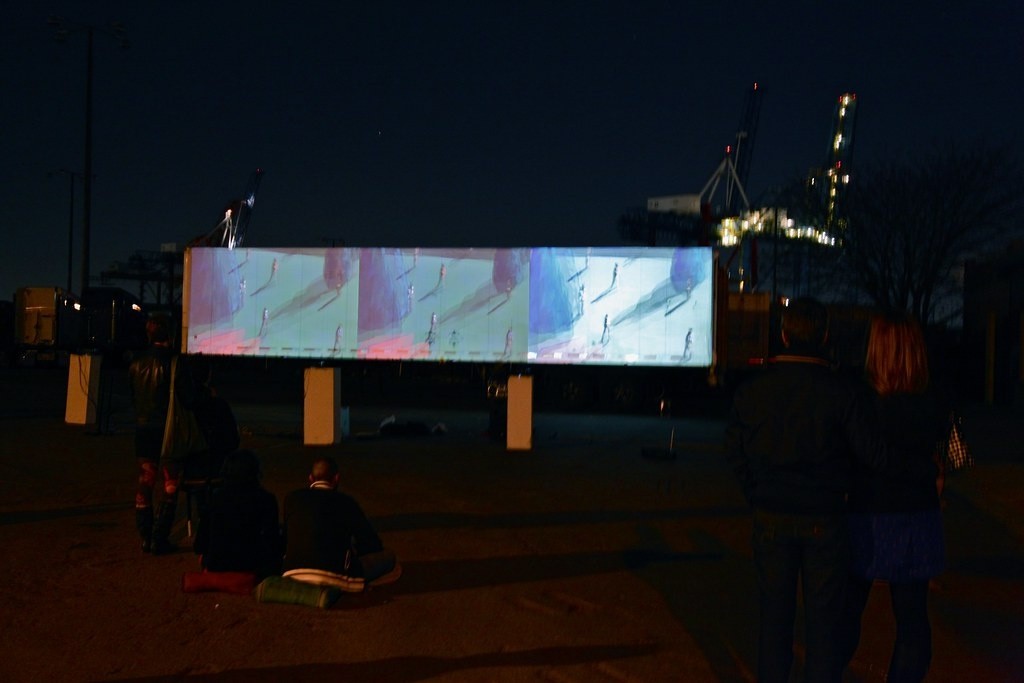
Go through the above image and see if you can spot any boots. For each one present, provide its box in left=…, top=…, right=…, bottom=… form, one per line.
left=150, top=512, right=178, bottom=556
left=135, top=509, right=155, bottom=553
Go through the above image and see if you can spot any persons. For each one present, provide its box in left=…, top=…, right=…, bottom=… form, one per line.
left=194, top=446, right=285, bottom=572
left=722, top=294, right=877, bottom=683
left=827, top=304, right=948, bottom=683
left=283, top=458, right=383, bottom=578
left=380, top=414, right=447, bottom=435
left=128, top=319, right=254, bottom=557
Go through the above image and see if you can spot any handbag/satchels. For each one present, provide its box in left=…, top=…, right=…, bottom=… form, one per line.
left=169, top=392, right=211, bottom=457
